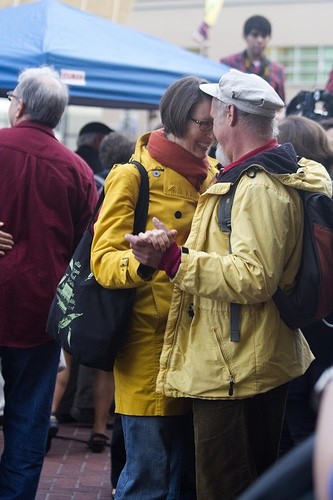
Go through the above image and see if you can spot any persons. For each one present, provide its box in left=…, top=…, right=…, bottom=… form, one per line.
left=0, top=66, right=100, bottom=500
left=124, top=70, right=333, bottom=500
left=38, top=120, right=136, bottom=456
left=219, top=15, right=287, bottom=105
left=0, top=221, right=14, bottom=257
left=90, top=75, right=220, bottom=500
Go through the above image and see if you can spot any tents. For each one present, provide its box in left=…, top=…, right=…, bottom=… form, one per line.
left=0, top=1, right=235, bottom=110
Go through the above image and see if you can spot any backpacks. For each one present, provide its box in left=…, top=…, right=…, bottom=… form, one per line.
left=218, top=172, right=333, bottom=329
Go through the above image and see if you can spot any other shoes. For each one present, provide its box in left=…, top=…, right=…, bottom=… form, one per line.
left=46, top=415, right=58, bottom=450
left=87, top=434, right=108, bottom=453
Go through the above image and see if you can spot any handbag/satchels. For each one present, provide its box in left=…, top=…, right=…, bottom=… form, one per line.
left=46, top=161, right=150, bottom=371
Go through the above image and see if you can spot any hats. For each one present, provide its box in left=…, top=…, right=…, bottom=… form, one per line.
left=199, top=70, right=284, bottom=119
left=79, top=122, right=112, bottom=134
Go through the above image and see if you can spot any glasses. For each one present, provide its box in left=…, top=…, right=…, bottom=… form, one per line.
left=189, top=115, right=213, bottom=131
left=7, top=91, right=20, bottom=101
